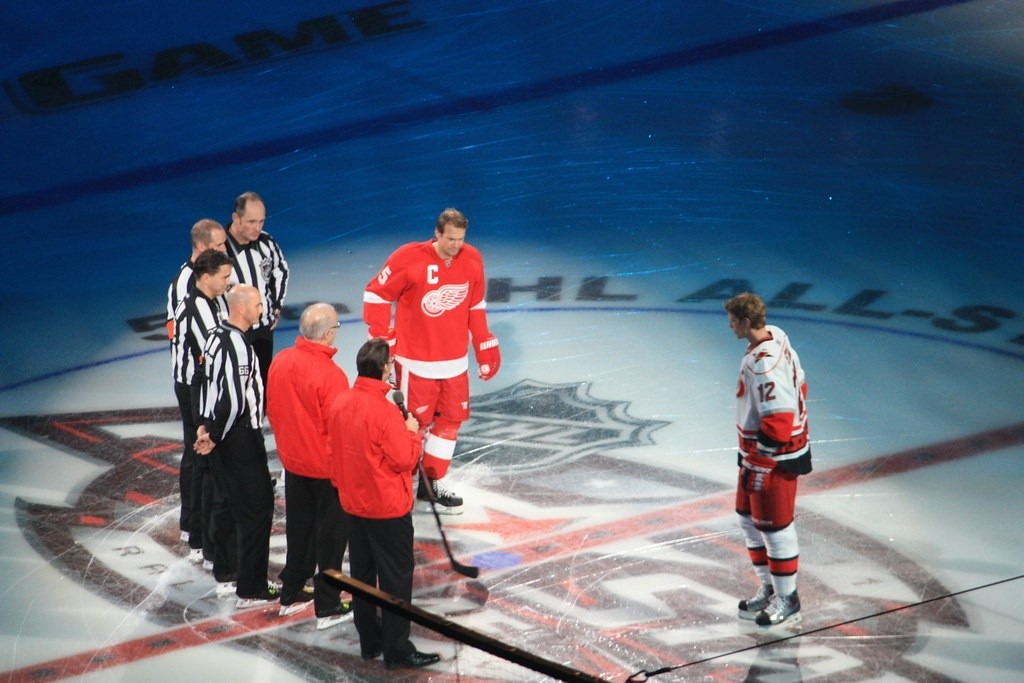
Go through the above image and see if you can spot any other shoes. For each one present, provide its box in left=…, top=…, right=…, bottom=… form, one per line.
left=188, top=548, right=203, bottom=566
left=181, top=531, right=190, bottom=542
left=203, top=559, right=215, bottom=571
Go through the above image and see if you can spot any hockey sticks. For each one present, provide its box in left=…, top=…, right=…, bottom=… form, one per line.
left=388, top=374, right=480, bottom=579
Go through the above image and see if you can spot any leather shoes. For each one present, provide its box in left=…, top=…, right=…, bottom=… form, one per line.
left=384, top=653, right=439, bottom=668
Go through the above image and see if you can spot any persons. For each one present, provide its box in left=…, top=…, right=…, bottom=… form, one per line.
left=192, top=284, right=282, bottom=608
left=167, top=217, right=232, bottom=342
left=263, top=303, right=356, bottom=631
left=169, top=249, right=233, bottom=570
left=362, top=205, right=501, bottom=514
left=325, top=337, right=441, bottom=668
left=223, top=191, right=285, bottom=414
left=724, top=289, right=813, bottom=628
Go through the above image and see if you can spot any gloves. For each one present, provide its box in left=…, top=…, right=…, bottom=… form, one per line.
left=472, top=332, right=501, bottom=380
left=368, top=328, right=398, bottom=361
left=744, top=454, right=777, bottom=492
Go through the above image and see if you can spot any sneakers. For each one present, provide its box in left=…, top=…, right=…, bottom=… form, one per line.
left=235, top=582, right=282, bottom=607
left=756, top=590, right=801, bottom=630
left=316, top=601, right=352, bottom=629
left=212, top=574, right=236, bottom=593
left=414, top=478, right=464, bottom=514
left=279, top=582, right=314, bottom=615
left=738, top=588, right=777, bottom=619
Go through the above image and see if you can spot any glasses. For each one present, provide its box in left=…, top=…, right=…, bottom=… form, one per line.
left=328, top=322, right=340, bottom=330
left=384, top=358, right=395, bottom=365
